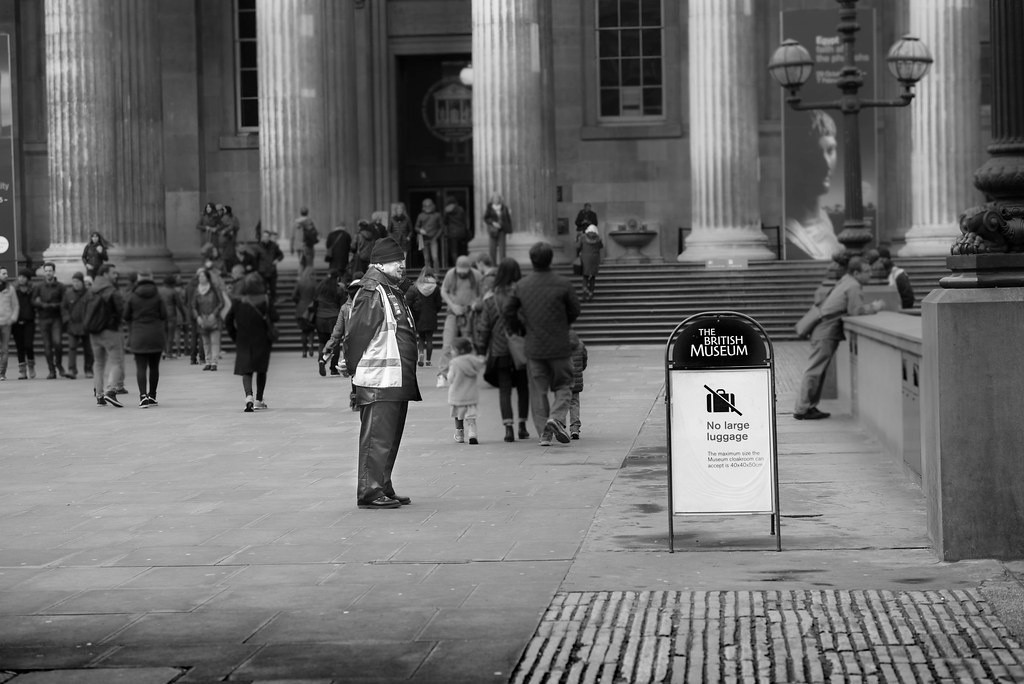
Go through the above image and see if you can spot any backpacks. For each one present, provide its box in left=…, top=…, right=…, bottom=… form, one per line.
left=79, top=286, right=116, bottom=337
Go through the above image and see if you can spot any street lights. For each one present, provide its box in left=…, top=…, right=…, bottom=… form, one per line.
left=767, top=0, right=933, bottom=285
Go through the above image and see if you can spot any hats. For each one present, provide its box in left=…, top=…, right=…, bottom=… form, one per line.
left=18, top=267, right=33, bottom=280
left=72, top=271, right=84, bottom=283
left=456, top=255, right=471, bottom=275
left=371, top=236, right=404, bottom=263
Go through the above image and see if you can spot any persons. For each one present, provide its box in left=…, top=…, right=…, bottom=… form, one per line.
left=290, top=206, right=318, bottom=275
left=326, top=196, right=469, bottom=271
left=84, top=263, right=126, bottom=408
left=436, top=242, right=588, bottom=447
left=124, top=271, right=166, bottom=407
left=293, top=266, right=364, bottom=412
left=0, top=262, right=94, bottom=380
left=887, top=265, right=916, bottom=309
left=792, top=257, right=883, bottom=420
left=195, top=202, right=240, bottom=271
left=483, top=193, right=514, bottom=262
left=785, top=108, right=840, bottom=258
left=405, top=265, right=442, bottom=366
left=157, top=257, right=225, bottom=370
left=576, top=201, right=604, bottom=300
left=228, top=222, right=285, bottom=413
left=82, top=231, right=109, bottom=281
left=335, top=237, right=422, bottom=510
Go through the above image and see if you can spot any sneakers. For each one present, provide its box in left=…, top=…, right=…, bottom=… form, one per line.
left=244, top=399, right=255, bottom=412
left=253, top=400, right=267, bottom=409
left=96, top=396, right=108, bottom=406
left=104, top=391, right=123, bottom=407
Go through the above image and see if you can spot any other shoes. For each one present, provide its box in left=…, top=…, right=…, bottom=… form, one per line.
left=454, top=415, right=584, bottom=445
left=203, top=363, right=210, bottom=370
left=114, top=389, right=130, bottom=394
left=793, top=407, right=831, bottom=420
left=418, top=353, right=424, bottom=367
left=211, top=365, right=216, bottom=370
left=0, top=360, right=95, bottom=379
left=148, top=396, right=158, bottom=405
left=425, top=361, right=431, bottom=367
left=319, top=360, right=326, bottom=376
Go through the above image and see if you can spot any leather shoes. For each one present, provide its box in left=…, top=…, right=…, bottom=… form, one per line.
left=359, top=495, right=402, bottom=509
left=390, top=494, right=411, bottom=505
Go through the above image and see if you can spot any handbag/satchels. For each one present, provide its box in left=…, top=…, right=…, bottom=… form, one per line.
left=202, top=316, right=218, bottom=331
left=796, top=303, right=823, bottom=339
left=506, top=334, right=527, bottom=370
left=264, top=315, right=280, bottom=341
left=573, top=256, right=582, bottom=276
